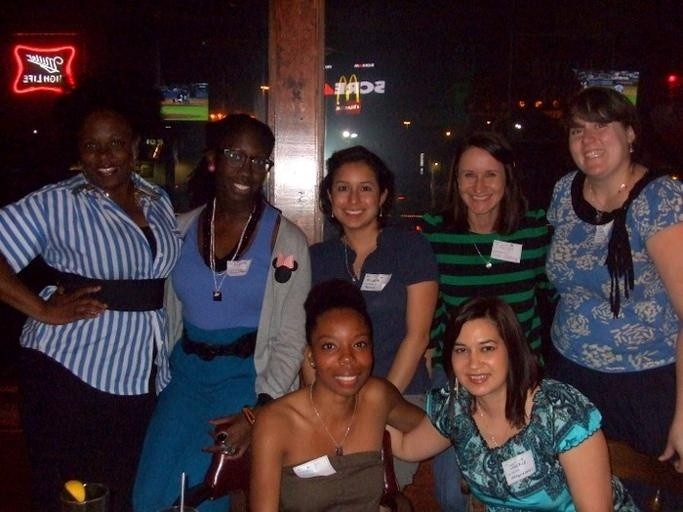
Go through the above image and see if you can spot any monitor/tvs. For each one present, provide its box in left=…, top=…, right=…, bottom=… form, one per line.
left=155, top=77, right=213, bottom=129
left=567, top=63, right=646, bottom=115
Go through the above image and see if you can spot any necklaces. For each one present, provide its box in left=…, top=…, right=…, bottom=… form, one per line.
left=474, top=399, right=511, bottom=448
left=204, top=198, right=266, bottom=301
left=588, top=164, right=637, bottom=224
left=309, top=384, right=358, bottom=456
left=342, top=233, right=377, bottom=284
left=467, top=224, right=494, bottom=269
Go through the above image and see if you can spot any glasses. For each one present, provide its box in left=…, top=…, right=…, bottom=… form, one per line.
left=212, top=141, right=275, bottom=175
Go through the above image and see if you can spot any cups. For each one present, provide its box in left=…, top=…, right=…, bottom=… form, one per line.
left=58, top=483, right=107, bottom=510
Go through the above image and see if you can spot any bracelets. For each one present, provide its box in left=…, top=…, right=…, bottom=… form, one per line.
left=242, top=407, right=256, bottom=428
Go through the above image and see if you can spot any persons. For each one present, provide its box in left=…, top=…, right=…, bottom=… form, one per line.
left=1, top=78, right=183, bottom=512
left=411, top=134, right=564, bottom=512
left=542, top=86, right=683, bottom=473
left=249, top=278, right=427, bottom=512
left=129, top=114, right=313, bottom=512
left=384, top=295, right=639, bottom=512
left=308, top=145, right=441, bottom=493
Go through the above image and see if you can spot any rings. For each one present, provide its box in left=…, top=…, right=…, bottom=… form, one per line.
left=223, top=446, right=235, bottom=455
left=216, top=432, right=229, bottom=445
